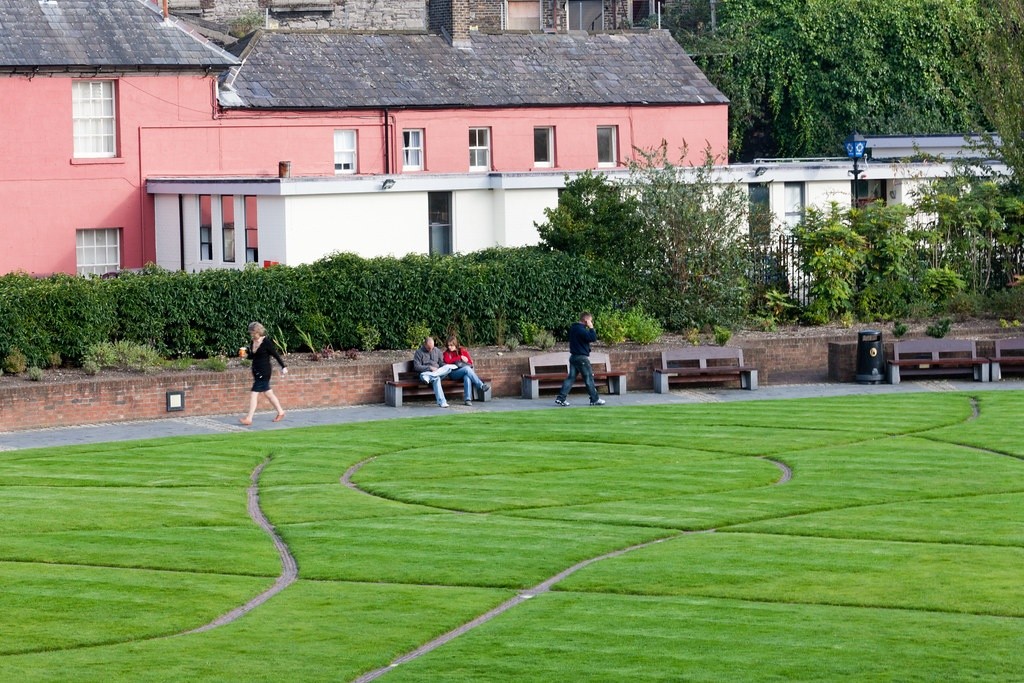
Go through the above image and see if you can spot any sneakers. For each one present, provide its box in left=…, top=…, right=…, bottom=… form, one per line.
left=422, top=376, right=430, bottom=384
left=590, top=399, right=606, bottom=405
left=555, top=400, right=570, bottom=406
left=439, top=403, right=449, bottom=408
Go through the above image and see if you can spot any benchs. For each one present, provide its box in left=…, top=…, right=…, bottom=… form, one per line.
left=887, top=340, right=989, bottom=385
left=521, top=352, right=627, bottom=399
left=988, top=338, right=1024, bottom=382
left=385, top=360, right=491, bottom=408
left=652, top=346, right=759, bottom=395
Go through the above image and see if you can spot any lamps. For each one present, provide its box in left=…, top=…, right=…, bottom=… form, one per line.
left=381, top=179, right=396, bottom=191
left=755, top=167, right=767, bottom=177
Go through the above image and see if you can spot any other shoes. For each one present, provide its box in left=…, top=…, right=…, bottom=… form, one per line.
left=239, top=418, right=252, bottom=425
left=272, top=414, right=285, bottom=422
left=482, top=385, right=491, bottom=392
left=465, top=401, right=472, bottom=406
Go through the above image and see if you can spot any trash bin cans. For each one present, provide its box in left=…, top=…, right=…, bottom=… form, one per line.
left=853, top=330, right=886, bottom=386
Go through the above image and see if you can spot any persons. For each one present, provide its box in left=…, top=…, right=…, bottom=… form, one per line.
left=414, top=337, right=452, bottom=408
left=555, top=312, right=605, bottom=406
left=239, top=322, right=288, bottom=426
left=444, top=336, right=491, bottom=406
